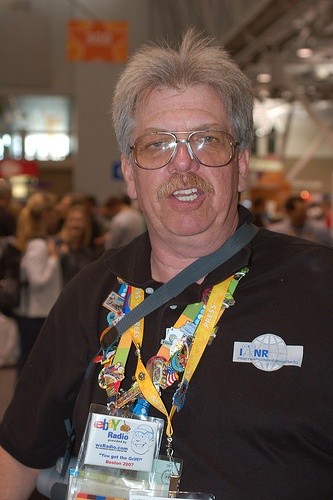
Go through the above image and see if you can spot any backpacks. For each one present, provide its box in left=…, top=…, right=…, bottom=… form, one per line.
left=0, top=232, right=48, bottom=312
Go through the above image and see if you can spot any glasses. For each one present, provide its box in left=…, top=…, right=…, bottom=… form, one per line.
left=127, top=129, right=243, bottom=170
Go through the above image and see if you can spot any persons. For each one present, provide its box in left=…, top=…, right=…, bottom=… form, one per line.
left=0, top=25, right=332, bottom=500
left=0, top=175, right=143, bottom=376
left=236, top=190, right=333, bottom=243
